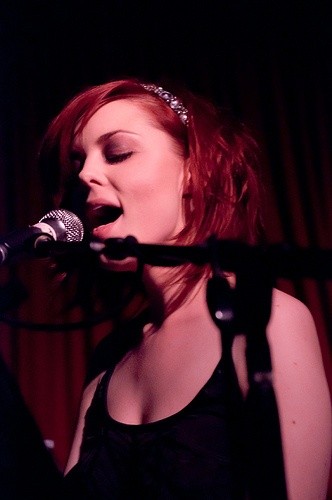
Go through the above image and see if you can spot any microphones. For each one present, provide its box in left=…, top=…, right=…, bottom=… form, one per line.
left=0, top=209, right=84, bottom=265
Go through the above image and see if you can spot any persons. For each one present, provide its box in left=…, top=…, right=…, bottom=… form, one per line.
left=0, top=79, right=331, bottom=499
